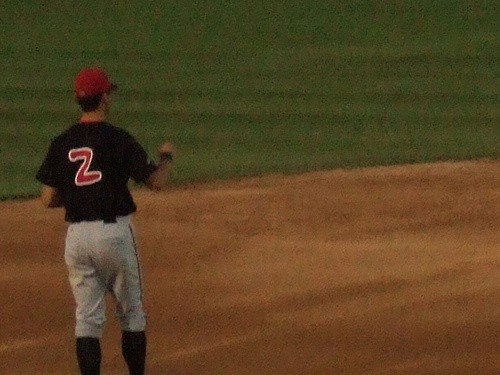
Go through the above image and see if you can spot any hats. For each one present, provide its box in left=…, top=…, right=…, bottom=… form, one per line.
left=72, top=67, right=118, bottom=98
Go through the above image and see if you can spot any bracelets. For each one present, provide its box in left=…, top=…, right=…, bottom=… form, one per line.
left=159, top=152, right=172, bottom=162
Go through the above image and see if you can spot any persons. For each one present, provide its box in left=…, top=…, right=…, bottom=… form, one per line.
left=35, top=65, right=181, bottom=375
